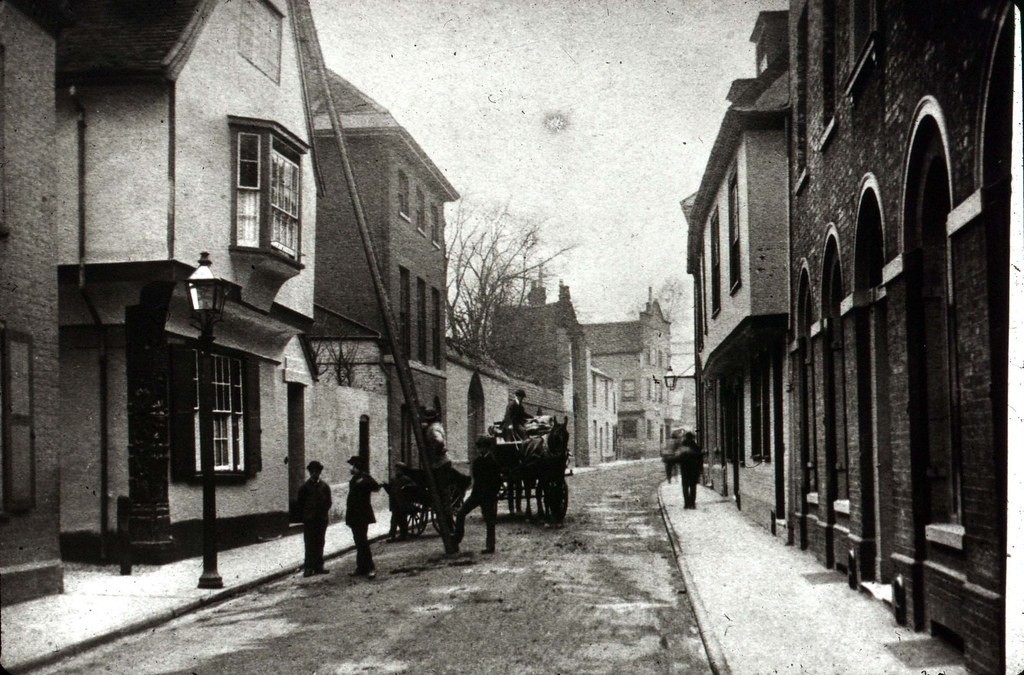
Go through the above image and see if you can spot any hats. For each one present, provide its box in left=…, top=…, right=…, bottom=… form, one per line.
left=513, top=388, right=526, bottom=399
left=345, top=456, right=366, bottom=471
left=474, top=436, right=492, bottom=447
left=306, top=461, right=324, bottom=471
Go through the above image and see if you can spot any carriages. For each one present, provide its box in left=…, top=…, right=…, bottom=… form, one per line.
left=490, top=414, right=573, bottom=526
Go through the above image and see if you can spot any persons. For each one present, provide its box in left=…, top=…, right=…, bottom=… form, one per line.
left=450, top=435, right=501, bottom=554
left=503, top=389, right=533, bottom=441
left=382, top=462, right=418, bottom=543
left=422, top=406, right=452, bottom=506
left=297, top=461, right=332, bottom=577
left=662, top=430, right=705, bottom=509
left=346, top=456, right=380, bottom=580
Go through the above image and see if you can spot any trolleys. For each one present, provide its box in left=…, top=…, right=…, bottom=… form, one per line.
left=406, top=461, right=471, bottom=539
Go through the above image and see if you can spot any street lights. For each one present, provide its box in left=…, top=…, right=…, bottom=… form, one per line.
left=188, top=252, right=224, bottom=589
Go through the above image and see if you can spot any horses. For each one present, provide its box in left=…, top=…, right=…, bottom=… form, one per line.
left=507, top=415, right=570, bottom=517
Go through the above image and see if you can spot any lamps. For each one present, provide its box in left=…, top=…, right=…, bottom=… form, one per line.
left=663, top=367, right=696, bottom=390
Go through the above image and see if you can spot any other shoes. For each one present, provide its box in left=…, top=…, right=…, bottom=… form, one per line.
left=314, top=565, right=329, bottom=575
left=303, top=568, right=313, bottom=577
left=367, top=568, right=376, bottom=577
left=481, top=547, right=495, bottom=554
left=347, top=568, right=363, bottom=577
